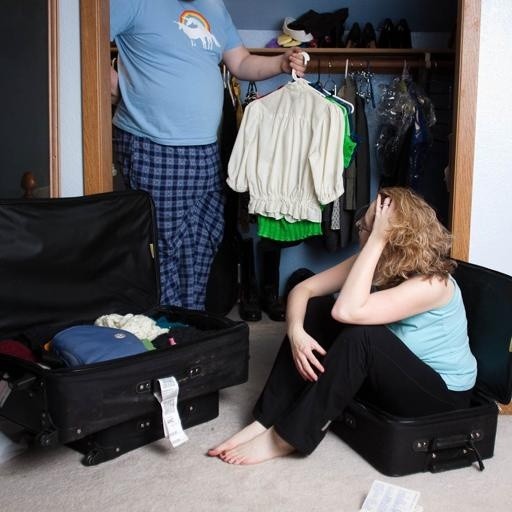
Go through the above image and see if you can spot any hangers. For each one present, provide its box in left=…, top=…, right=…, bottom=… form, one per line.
left=111, top=52, right=442, bottom=117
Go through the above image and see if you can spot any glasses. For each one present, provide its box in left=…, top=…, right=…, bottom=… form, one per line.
left=355, top=217, right=371, bottom=233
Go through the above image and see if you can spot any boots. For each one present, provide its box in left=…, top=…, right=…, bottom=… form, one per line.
left=257, top=238, right=285, bottom=321
left=239, top=238, right=261, bottom=321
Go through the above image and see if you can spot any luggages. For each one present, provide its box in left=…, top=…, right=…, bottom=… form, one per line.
left=0, top=190, right=247, bottom=466
left=329, top=254, right=512, bottom=477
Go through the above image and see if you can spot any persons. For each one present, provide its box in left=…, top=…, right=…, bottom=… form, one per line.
left=206, top=185, right=479, bottom=465
left=111, top=0, right=309, bottom=311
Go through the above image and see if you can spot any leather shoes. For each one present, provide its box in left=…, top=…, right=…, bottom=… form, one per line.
left=347, top=18, right=412, bottom=48
